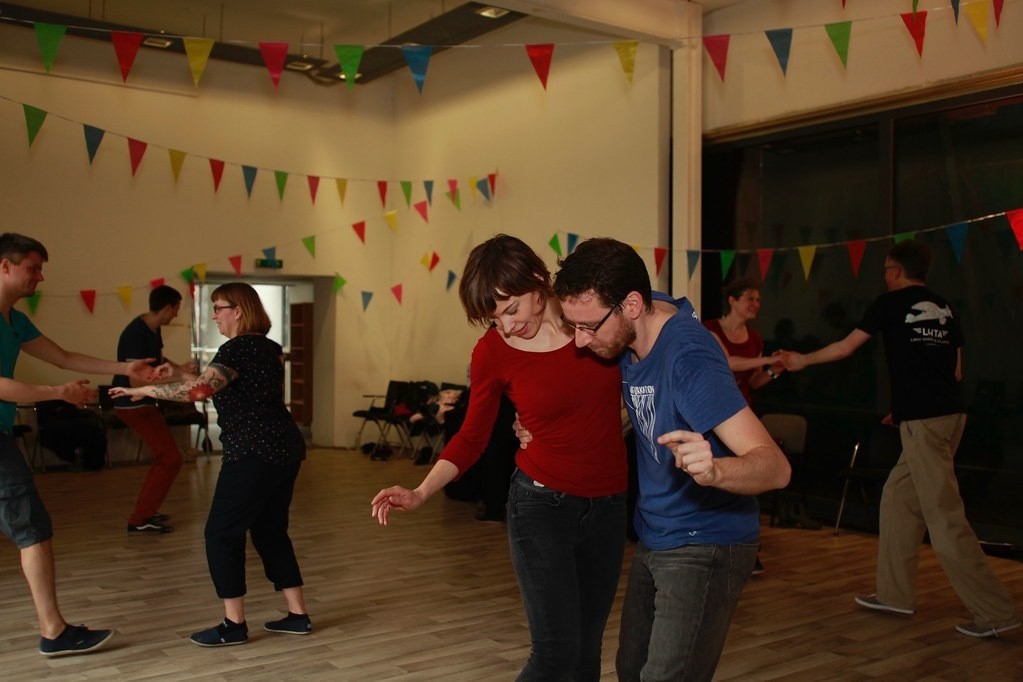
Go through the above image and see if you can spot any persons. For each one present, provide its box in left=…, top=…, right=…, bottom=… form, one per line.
left=512, top=237, right=792, bottom=682
left=370, top=232, right=628, bottom=682
left=703, top=276, right=786, bottom=407
left=780, top=237, right=1022, bottom=635
left=111, top=286, right=181, bottom=534
left=0, top=232, right=166, bottom=656
left=108, top=283, right=311, bottom=646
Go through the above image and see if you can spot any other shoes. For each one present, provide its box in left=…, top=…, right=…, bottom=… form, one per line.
left=855, top=594, right=915, bottom=614
left=955, top=615, right=1023, bottom=637
left=750, top=559, right=765, bottom=574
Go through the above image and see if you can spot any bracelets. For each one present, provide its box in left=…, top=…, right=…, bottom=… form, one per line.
left=767, top=368, right=777, bottom=379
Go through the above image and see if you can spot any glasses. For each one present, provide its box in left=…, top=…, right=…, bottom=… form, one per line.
left=881, top=265, right=899, bottom=274
left=561, top=291, right=632, bottom=336
left=213, top=304, right=236, bottom=314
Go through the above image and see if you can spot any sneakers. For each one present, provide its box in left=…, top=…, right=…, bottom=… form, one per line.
left=147, top=511, right=170, bottom=523
left=265, top=611, right=312, bottom=634
left=129, top=524, right=174, bottom=535
left=40, top=621, right=112, bottom=656
left=188, top=620, right=250, bottom=646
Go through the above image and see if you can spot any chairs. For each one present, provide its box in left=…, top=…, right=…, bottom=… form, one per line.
left=13, top=385, right=211, bottom=474
left=759, top=414, right=809, bottom=529
left=834, top=428, right=903, bottom=536
left=353, top=381, right=467, bottom=463
